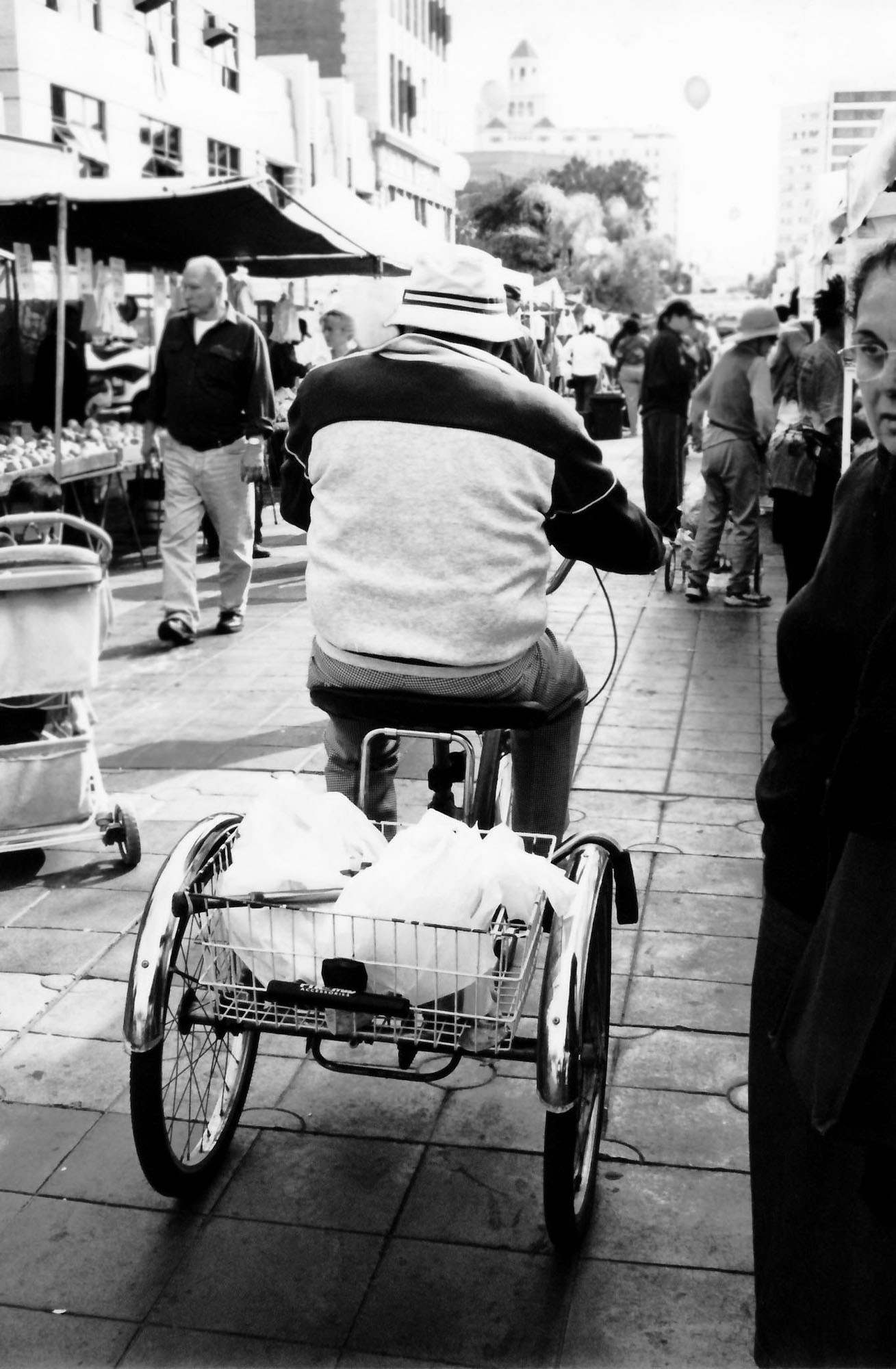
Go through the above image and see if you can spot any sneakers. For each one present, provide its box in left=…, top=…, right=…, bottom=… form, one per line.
left=685, top=580, right=708, bottom=598
left=724, top=586, right=771, bottom=606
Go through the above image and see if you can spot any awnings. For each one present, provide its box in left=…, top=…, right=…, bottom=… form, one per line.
left=0, top=175, right=412, bottom=278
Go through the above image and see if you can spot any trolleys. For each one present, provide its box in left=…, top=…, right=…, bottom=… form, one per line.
left=663, top=442, right=767, bottom=597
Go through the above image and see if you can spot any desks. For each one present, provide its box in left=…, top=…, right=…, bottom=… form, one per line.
left=0, top=439, right=277, bottom=568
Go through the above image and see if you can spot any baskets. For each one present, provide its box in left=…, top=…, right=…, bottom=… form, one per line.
left=174, top=814, right=560, bottom=1051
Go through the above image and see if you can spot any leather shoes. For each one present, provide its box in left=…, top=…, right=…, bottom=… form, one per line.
left=158, top=618, right=193, bottom=644
left=217, top=612, right=243, bottom=632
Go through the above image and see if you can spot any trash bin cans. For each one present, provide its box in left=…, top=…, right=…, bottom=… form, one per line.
left=582, top=391, right=627, bottom=441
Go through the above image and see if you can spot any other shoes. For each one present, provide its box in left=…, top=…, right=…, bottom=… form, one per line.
left=252, top=543, right=271, bottom=558
left=196, top=553, right=220, bottom=563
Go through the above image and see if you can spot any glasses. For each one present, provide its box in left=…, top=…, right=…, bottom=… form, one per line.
left=836, top=343, right=896, bottom=380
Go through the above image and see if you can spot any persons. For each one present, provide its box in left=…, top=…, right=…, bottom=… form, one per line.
left=319, top=309, right=363, bottom=362
left=278, top=243, right=673, bottom=855
left=140, top=255, right=277, bottom=645
left=749, top=241, right=895, bottom=1369
left=0, top=473, right=64, bottom=880
left=501, top=274, right=844, bottom=607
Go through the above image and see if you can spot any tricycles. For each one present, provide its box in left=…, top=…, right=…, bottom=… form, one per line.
left=121, top=554, right=641, bottom=1249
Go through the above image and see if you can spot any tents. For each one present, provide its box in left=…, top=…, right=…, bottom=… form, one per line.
left=770, top=103, right=895, bottom=475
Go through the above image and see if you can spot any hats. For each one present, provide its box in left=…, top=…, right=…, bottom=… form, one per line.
left=503, top=284, right=521, bottom=302
left=666, top=300, right=706, bottom=320
left=721, top=307, right=800, bottom=342
left=384, top=242, right=528, bottom=341
